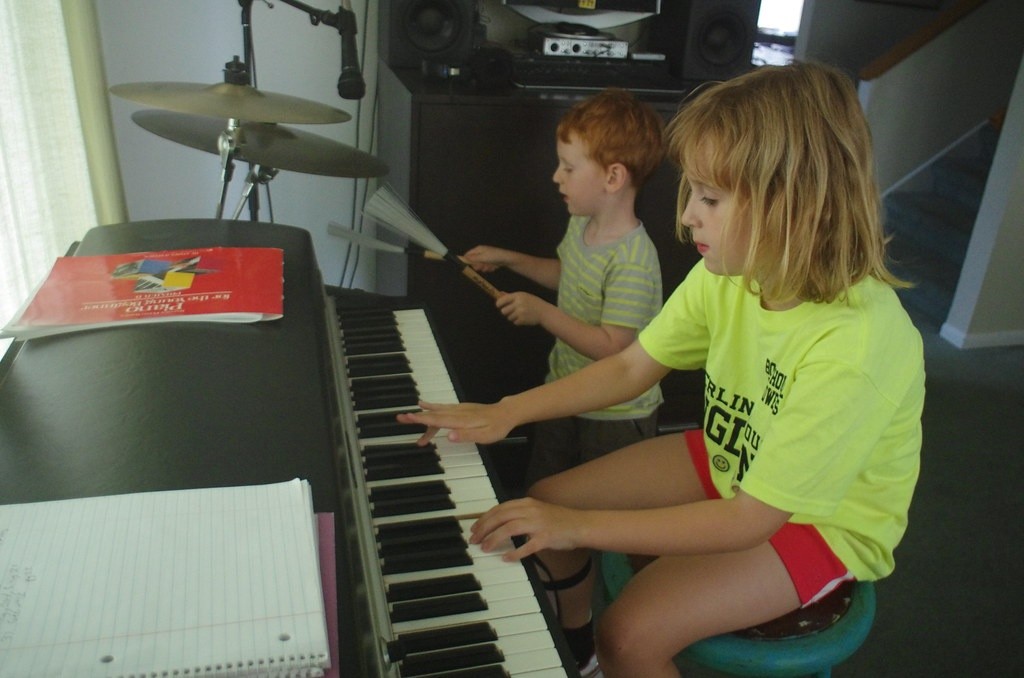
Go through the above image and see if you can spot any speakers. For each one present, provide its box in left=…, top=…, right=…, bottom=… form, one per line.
left=379, top=0, right=490, bottom=98
left=647, top=0, right=762, bottom=92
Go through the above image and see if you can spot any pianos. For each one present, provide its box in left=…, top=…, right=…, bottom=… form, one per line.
left=0, top=205, right=587, bottom=678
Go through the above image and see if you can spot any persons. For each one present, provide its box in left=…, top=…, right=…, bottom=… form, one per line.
left=397, top=62, right=925, bottom=678
left=464, top=92, right=665, bottom=599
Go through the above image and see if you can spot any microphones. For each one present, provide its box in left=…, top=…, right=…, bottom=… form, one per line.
left=337, top=0, right=366, bottom=100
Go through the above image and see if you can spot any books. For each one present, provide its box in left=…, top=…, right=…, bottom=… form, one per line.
left=0, top=478, right=340, bottom=678
left=1, top=247, right=284, bottom=341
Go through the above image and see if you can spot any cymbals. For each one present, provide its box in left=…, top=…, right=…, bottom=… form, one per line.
left=110, top=82, right=355, bottom=126
left=129, top=110, right=389, bottom=178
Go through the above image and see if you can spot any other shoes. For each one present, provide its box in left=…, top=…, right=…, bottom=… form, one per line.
left=564, top=623, right=599, bottom=675
